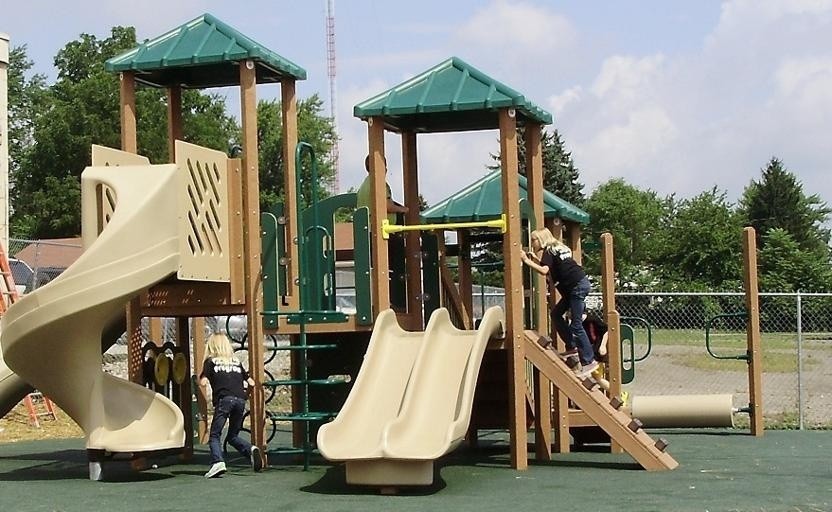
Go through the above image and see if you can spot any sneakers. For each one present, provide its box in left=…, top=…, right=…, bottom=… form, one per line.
left=250, top=446, right=262, bottom=471
left=560, top=349, right=578, bottom=357
left=204, top=461, right=225, bottom=478
left=576, top=359, right=599, bottom=377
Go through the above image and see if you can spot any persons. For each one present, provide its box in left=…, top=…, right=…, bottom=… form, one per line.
left=577, top=311, right=630, bottom=410
left=518, top=226, right=601, bottom=378
left=355, top=152, right=412, bottom=315
left=197, top=332, right=268, bottom=479
